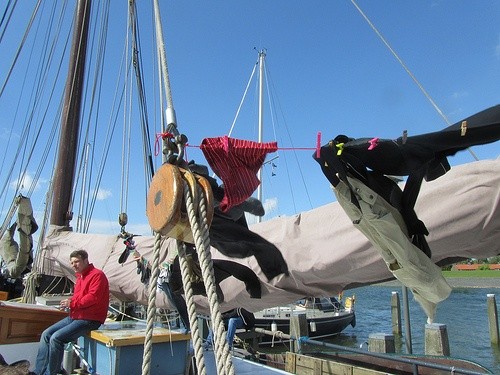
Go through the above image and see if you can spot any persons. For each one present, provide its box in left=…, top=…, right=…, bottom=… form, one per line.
left=201, top=308, right=255, bottom=352
left=26, top=249, right=109, bottom=375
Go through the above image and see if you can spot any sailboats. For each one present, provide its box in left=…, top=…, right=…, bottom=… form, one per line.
left=0, top=1, right=494, bottom=375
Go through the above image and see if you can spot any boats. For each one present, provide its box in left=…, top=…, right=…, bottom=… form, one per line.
left=228, top=290, right=357, bottom=348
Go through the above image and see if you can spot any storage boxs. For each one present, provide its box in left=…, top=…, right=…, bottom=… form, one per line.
left=76, top=320, right=190, bottom=374
